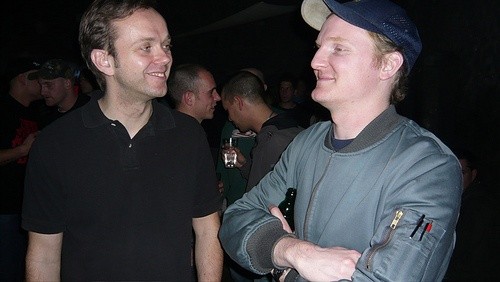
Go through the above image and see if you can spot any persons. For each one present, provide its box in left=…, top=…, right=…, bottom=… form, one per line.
left=0, top=65, right=500, bottom=282
left=21, top=0, right=223, bottom=282
left=216, top=0, right=464, bottom=282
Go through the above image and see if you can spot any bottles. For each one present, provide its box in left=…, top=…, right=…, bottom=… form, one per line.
left=278, top=188, right=298, bottom=232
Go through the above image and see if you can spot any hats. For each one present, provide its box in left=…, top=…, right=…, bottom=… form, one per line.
left=28, top=59, right=73, bottom=80
left=301, top=0, right=421, bottom=76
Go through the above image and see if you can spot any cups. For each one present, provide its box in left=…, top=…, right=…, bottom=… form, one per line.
left=223, top=136, right=239, bottom=168
